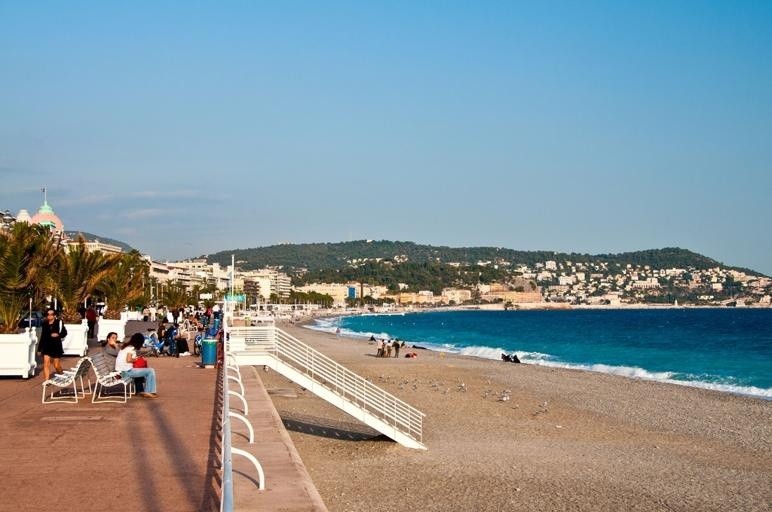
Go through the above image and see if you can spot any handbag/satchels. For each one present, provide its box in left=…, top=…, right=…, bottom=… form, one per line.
left=133, top=358, right=147, bottom=368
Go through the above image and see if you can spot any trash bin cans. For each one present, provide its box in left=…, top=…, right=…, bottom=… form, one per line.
left=202, top=339, right=218, bottom=368
left=214, top=319, right=219, bottom=331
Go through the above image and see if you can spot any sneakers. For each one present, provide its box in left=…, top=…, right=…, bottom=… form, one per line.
left=136, top=392, right=160, bottom=398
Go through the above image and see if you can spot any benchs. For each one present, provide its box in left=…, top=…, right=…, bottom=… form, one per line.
left=40, top=352, right=138, bottom=405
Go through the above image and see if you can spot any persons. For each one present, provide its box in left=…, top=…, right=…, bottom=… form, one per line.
left=336, top=327, right=341, bottom=339
left=115, top=337, right=160, bottom=398
left=36, top=308, right=67, bottom=386
left=104, top=332, right=145, bottom=393
left=137, top=303, right=224, bottom=358
left=369, top=334, right=413, bottom=358
left=79, top=302, right=86, bottom=319
left=86, top=303, right=98, bottom=339
left=502, top=353, right=520, bottom=363
